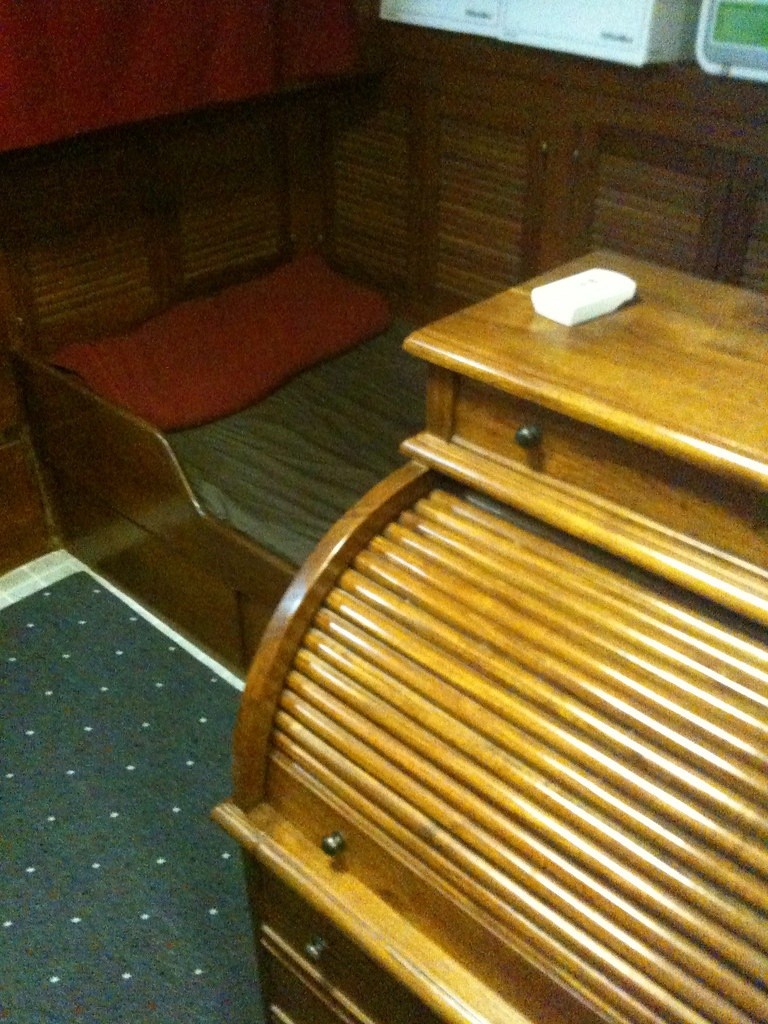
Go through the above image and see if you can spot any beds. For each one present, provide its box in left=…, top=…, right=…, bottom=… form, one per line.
left=22, top=256, right=484, bottom=686
